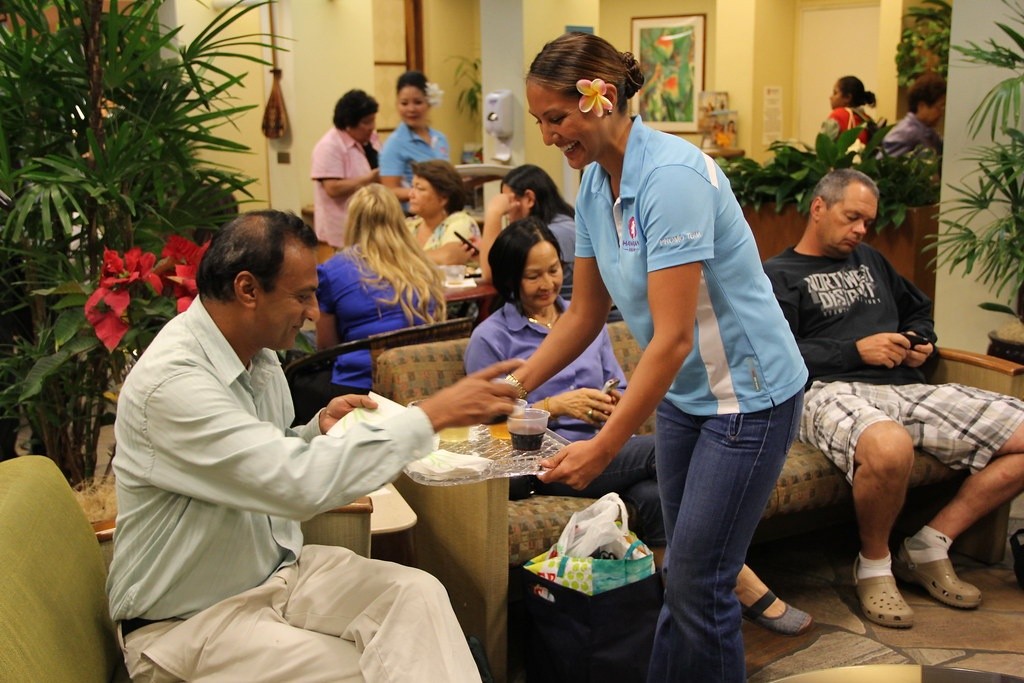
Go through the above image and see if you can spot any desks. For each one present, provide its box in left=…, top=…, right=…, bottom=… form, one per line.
left=441, top=285, right=499, bottom=333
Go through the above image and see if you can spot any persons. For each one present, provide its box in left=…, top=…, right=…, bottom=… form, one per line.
left=876, top=76, right=946, bottom=180
left=294, top=70, right=577, bottom=414
left=703, top=101, right=738, bottom=147
left=104, top=208, right=526, bottom=683
left=493, top=30, right=809, bottom=683
left=463, top=216, right=815, bottom=637
left=818, top=76, right=880, bottom=167
left=764, top=169, right=1024, bottom=629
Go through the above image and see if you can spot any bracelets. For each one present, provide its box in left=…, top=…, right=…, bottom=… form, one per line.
left=504, top=373, right=528, bottom=399
left=544, top=396, right=559, bottom=422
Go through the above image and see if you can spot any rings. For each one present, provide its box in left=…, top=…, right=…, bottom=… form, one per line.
left=587, top=409, right=594, bottom=417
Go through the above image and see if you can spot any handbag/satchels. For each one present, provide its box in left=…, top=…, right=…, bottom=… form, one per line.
left=509, top=491, right=661, bottom=682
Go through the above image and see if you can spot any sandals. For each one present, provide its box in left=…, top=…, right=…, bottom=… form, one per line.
left=898, top=538, right=983, bottom=609
left=854, top=557, right=915, bottom=626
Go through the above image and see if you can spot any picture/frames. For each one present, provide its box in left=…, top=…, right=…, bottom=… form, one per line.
left=629, top=12, right=708, bottom=133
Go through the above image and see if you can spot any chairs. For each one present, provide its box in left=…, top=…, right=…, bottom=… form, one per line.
left=287, top=301, right=481, bottom=428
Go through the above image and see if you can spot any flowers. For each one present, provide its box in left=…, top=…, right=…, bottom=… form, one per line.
left=85, top=237, right=214, bottom=354
left=423, top=82, right=445, bottom=108
left=575, top=79, right=614, bottom=116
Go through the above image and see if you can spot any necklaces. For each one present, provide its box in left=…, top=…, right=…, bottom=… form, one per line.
left=525, top=309, right=557, bottom=328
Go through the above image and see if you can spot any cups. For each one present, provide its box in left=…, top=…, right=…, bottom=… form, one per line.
left=440, top=426, right=470, bottom=442
left=490, top=398, right=527, bottom=439
left=445, top=265, right=465, bottom=284
left=484, top=379, right=516, bottom=425
left=509, top=408, right=551, bottom=453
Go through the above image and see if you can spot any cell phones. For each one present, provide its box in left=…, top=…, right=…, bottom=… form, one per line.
left=454, top=231, right=480, bottom=256
left=602, top=378, right=620, bottom=394
left=898, top=332, right=929, bottom=352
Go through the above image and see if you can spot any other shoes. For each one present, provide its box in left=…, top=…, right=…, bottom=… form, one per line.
left=743, top=590, right=811, bottom=637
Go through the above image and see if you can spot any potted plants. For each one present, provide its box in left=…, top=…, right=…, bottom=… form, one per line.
left=918, top=0, right=1024, bottom=374
left=0, top=0, right=277, bottom=561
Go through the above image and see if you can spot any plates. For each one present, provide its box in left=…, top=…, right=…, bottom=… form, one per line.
left=455, top=165, right=514, bottom=177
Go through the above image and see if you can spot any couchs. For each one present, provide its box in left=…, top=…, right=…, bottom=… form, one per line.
left=0, top=452, right=373, bottom=681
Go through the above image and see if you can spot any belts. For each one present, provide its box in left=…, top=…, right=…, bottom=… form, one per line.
left=121, top=615, right=161, bottom=637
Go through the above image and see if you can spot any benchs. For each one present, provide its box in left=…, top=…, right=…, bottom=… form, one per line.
left=377, top=322, right=1024, bottom=632
